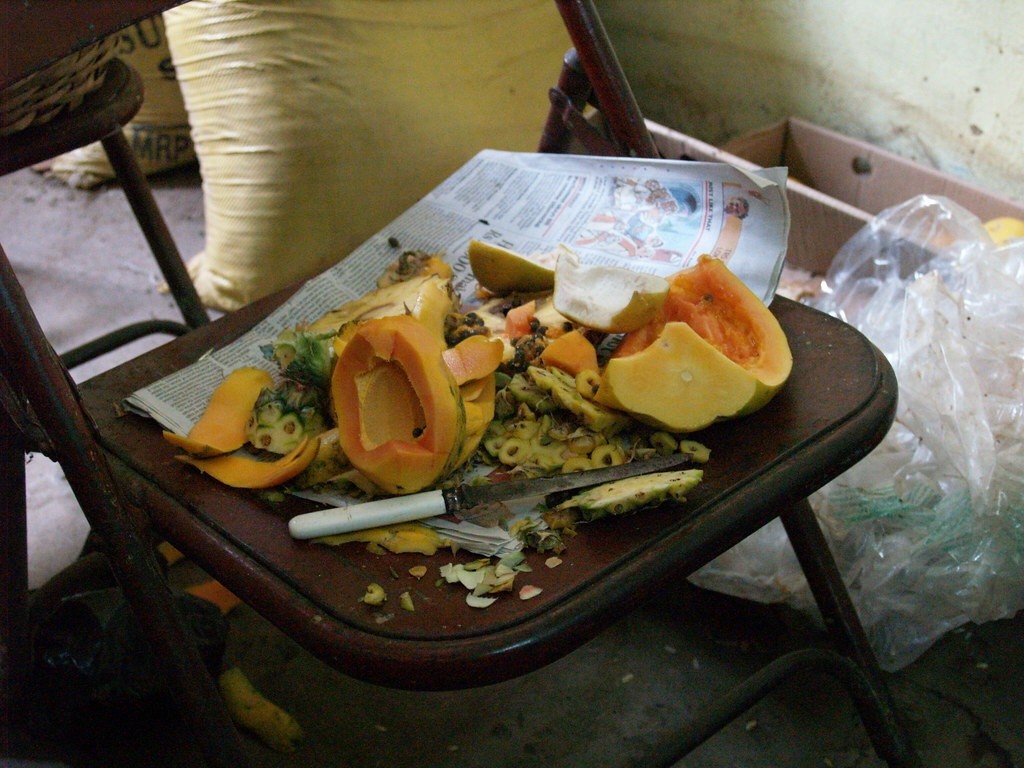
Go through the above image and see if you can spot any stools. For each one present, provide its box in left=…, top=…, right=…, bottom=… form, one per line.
left=2, top=57, right=214, bottom=598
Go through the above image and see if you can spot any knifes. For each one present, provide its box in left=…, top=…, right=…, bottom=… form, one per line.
left=287, top=453, right=693, bottom=542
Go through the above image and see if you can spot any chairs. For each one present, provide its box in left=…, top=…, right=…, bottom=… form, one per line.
left=0, top=0, right=924, bottom=767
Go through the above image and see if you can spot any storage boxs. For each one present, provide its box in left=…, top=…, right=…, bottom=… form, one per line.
left=720, top=111, right=1024, bottom=303
